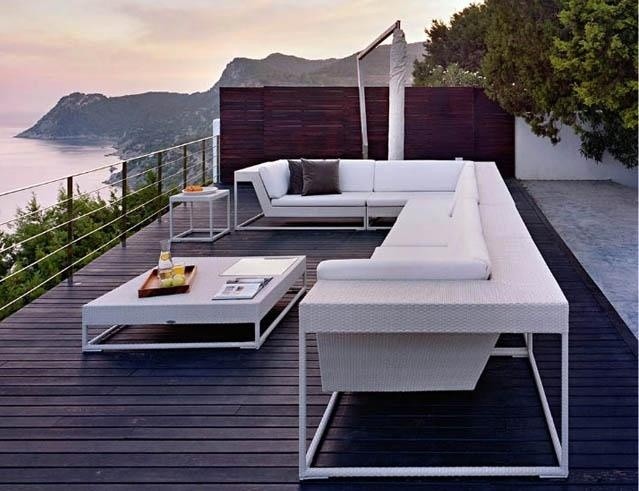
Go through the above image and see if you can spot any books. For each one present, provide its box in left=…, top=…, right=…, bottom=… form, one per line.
left=211, top=276, right=273, bottom=300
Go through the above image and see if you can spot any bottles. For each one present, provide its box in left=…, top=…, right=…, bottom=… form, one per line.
left=158, top=239, right=173, bottom=285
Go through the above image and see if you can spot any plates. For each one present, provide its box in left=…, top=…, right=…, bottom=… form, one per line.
left=182, top=187, right=218, bottom=193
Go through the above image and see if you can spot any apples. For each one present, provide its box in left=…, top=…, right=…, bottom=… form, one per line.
left=172, top=275, right=185, bottom=286
left=160, top=277, right=173, bottom=287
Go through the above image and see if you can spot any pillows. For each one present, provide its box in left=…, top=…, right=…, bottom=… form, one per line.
left=301, top=160, right=342, bottom=197
left=288, top=159, right=303, bottom=195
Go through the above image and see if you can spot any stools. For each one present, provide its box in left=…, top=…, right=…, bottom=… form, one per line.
left=170, top=187, right=230, bottom=242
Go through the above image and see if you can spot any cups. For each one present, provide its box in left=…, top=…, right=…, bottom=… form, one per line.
left=173, top=266, right=186, bottom=286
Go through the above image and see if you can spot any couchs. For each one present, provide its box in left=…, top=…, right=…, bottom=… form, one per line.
left=298, top=198, right=570, bottom=480
left=233, top=160, right=515, bottom=231
